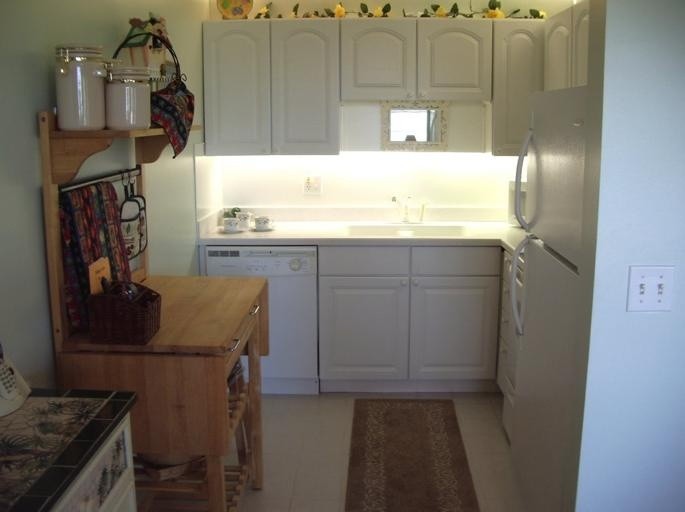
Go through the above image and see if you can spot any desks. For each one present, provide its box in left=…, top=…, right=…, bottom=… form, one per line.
left=55, top=267, right=269, bottom=512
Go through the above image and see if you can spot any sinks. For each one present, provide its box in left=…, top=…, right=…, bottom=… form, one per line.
left=345, top=225, right=467, bottom=237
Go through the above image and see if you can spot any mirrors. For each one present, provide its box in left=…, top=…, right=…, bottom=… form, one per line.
left=381, top=100, right=448, bottom=150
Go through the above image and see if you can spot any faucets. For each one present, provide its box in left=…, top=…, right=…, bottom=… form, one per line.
left=403, top=192, right=414, bottom=223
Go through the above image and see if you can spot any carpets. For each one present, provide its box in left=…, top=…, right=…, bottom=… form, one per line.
left=346, top=396, right=479, bottom=511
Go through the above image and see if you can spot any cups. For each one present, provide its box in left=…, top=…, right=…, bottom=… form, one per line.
left=236, top=212, right=252, bottom=226
left=254, top=216, right=271, bottom=229
left=224, top=218, right=238, bottom=231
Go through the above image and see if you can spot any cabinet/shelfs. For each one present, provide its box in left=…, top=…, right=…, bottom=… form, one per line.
left=319, top=248, right=501, bottom=393
left=342, top=16, right=491, bottom=101
left=203, top=18, right=342, bottom=156
left=544, top=3, right=589, bottom=89
left=484, top=18, right=544, bottom=157
left=496, top=250, right=524, bottom=425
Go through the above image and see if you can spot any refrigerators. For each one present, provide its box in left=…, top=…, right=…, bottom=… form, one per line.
left=507, top=87, right=599, bottom=511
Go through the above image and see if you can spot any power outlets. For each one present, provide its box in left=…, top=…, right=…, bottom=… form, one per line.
left=625, top=267, right=674, bottom=312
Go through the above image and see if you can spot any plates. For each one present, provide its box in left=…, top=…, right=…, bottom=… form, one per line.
left=219, top=224, right=274, bottom=233
